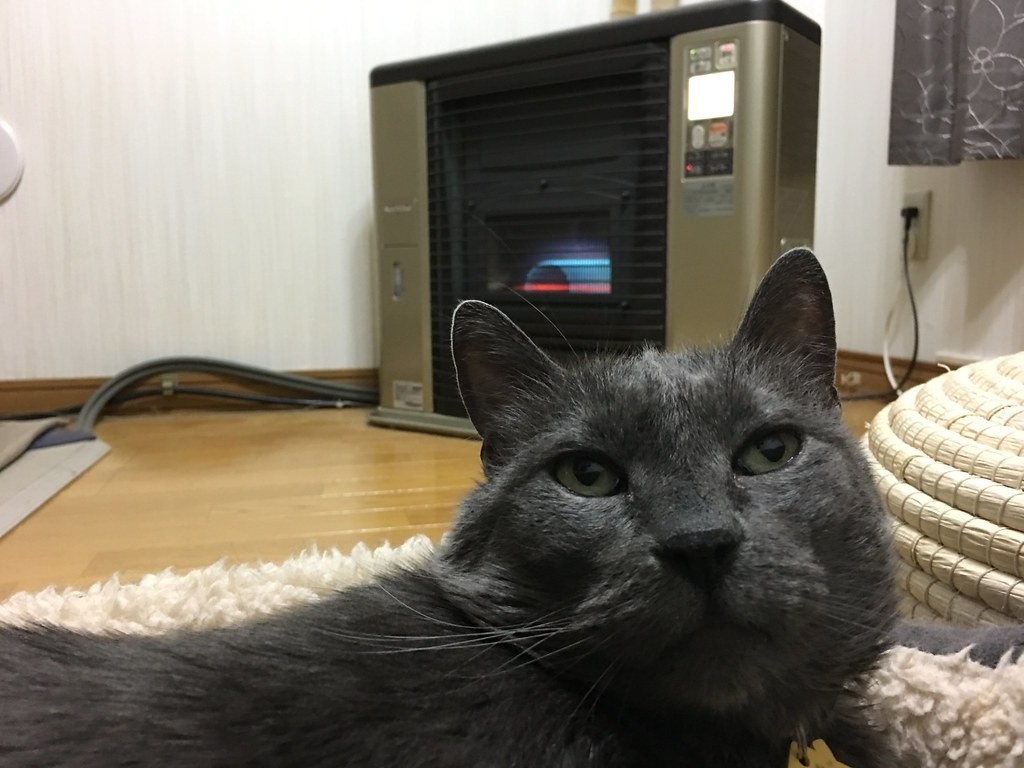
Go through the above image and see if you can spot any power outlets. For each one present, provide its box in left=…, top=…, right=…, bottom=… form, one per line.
left=901, top=190, right=933, bottom=261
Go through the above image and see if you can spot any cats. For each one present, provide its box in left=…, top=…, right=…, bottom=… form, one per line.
left=0, top=246, right=902, bottom=767
